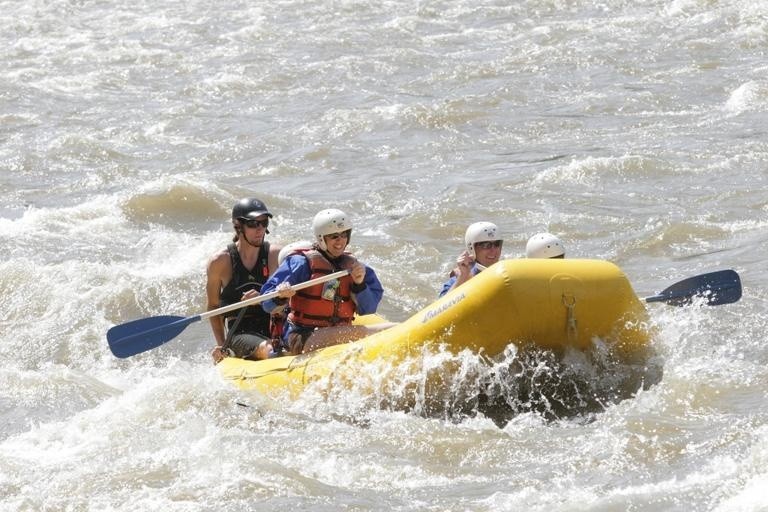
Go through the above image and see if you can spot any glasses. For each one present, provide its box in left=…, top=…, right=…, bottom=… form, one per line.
left=328, top=232, right=349, bottom=239
left=477, top=242, right=501, bottom=248
left=246, top=219, right=268, bottom=228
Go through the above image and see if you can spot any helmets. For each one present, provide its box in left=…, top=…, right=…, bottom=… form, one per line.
left=313, top=209, right=352, bottom=251
left=526, top=233, right=565, bottom=258
left=232, top=198, right=273, bottom=221
left=465, top=222, right=501, bottom=261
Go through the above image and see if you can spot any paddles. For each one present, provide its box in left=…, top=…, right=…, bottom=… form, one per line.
left=639, top=269, right=742, bottom=309
left=107, top=268, right=352, bottom=358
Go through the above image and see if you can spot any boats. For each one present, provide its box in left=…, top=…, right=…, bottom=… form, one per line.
left=214, top=257, right=652, bottom=408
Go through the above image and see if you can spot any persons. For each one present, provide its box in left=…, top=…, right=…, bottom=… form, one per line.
left=260, top=208, right=399, bottom=354
left=207, top=198, right=286, bottom=365
left=437, top=221, right=504, bottom=303
left=527, top=232, right=566, bottom=261
